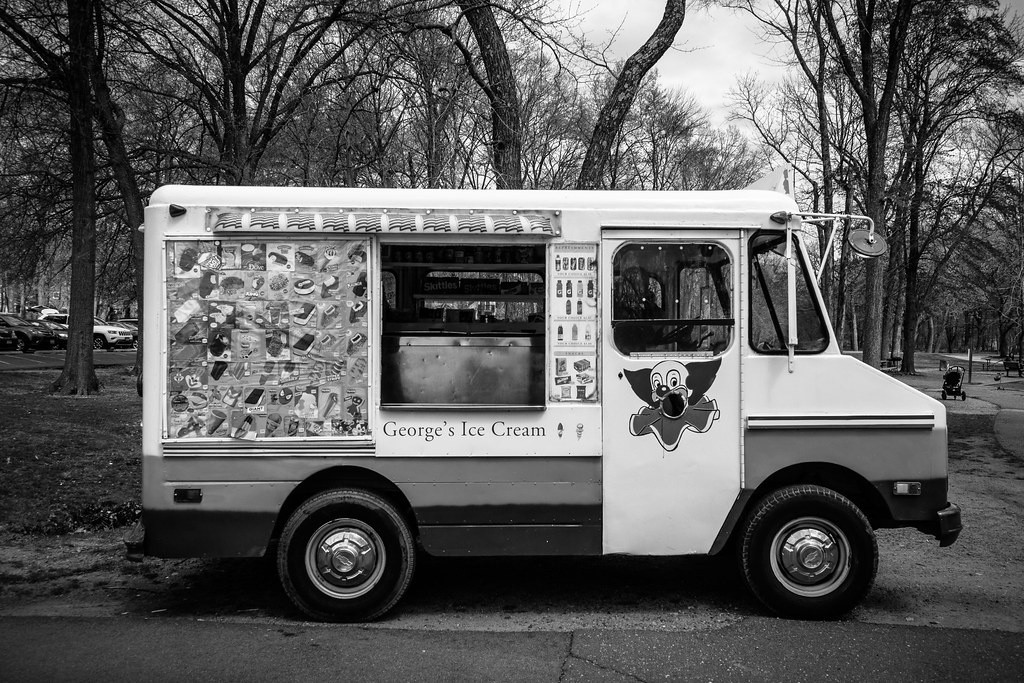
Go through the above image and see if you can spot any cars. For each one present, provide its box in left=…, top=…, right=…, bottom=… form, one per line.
left=0, top=305, right=136, bottom=353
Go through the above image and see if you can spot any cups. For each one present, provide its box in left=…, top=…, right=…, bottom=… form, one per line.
left=208, top=409, right=227, bottom=435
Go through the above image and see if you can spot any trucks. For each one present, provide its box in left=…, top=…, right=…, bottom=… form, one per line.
left=139, top=184, right=963, bottom=620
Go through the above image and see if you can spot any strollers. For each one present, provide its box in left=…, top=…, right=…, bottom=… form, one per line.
left=942, top=365, right=967, bottom=401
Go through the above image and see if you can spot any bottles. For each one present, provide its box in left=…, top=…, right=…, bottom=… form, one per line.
left=442, top=307, right=446, bottom=322
left=588, top=280, right=593, bottom=297
left=566, top=280, right=572, bottom=297
left=572, top=324, right=578, bottom=340
left=585, top=331, right=591, bottom=343
left=587, top=257, right=592, bottom=270
left=566, top=300, right=571, bottom=314
left=556, top=255, right=561, bottom=270
left=485, top=315, right=488, bottom=323
left=577, top=301, right=582, bottom=314
left=558, top=325, right=563, bottom=341
left=578, top=281, right=583, bottom=297
left=557, top=280, right=562, bottom=297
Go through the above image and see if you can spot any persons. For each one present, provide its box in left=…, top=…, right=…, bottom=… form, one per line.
left=613, top=266, right=667, bottom=353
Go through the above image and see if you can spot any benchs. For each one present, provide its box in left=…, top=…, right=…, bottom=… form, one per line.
left=1003, top=361, right=1021, bottom=378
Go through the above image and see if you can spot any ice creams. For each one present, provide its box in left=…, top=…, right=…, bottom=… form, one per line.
left=264, top=413, right=282, bottom=437
